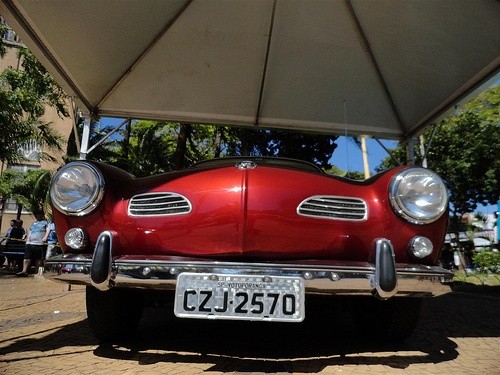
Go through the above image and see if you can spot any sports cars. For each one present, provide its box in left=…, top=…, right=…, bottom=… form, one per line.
left=42, top=156, right=454, bottom=324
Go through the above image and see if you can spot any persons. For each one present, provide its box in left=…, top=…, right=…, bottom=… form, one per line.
left=0, top=210, right=57, bottom=277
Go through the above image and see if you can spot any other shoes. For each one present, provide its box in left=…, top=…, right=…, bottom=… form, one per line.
left=16, top=272, right=28, bottom=276
